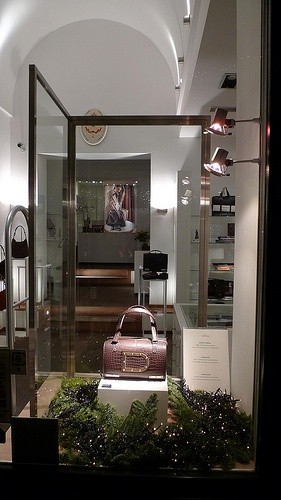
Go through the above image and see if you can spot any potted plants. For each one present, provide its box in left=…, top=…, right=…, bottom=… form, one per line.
left=135, top=230, right=150, bottom=241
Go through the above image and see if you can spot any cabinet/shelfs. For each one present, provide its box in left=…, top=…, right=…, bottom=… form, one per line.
left=191, top=212, right=236, bottom=302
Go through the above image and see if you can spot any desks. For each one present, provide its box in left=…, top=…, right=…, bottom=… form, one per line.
left=139, top=265, right=169, bottom=314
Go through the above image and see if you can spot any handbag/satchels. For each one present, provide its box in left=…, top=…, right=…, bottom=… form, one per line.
left=211, top=187, right=235, bottom=216
left=106, top=210, right=126, bottom=227
left=102, top=305, right=168, bottom=380
left=143, top=249, right=168, bottom=272
left=10, top=225, right=29, bottom=258
left=0, top=245, right=5, bottom=281
left=0, top=282, right=6, bottom=311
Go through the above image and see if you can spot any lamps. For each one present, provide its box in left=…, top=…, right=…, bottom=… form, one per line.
left=203, top=107, right=263, bottom=137
left=175, top=85, right=180, bottom=90
left=179, top=56, right=183, bottom=63
left=184, top=16, right=190, bottom=24
left=181, top=189, right=192, bottom=205
left=203, top=147, right=263, bottom=177
left=182, top=176, right=190, bottom=185
